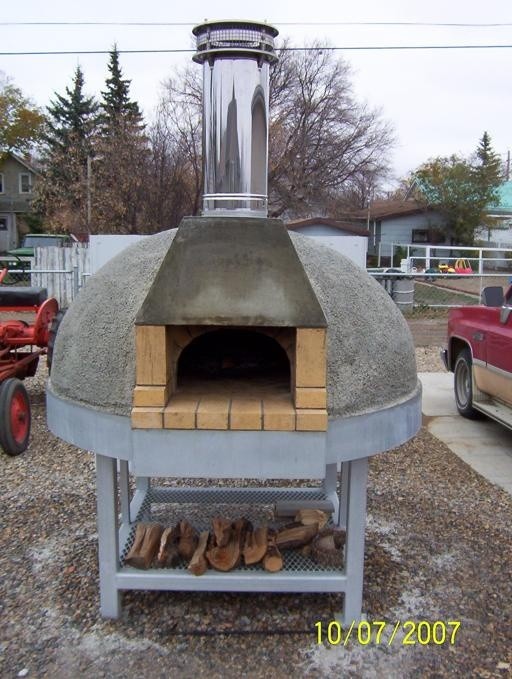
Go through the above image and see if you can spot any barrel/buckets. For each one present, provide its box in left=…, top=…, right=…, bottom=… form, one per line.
left=401, top=259, right=411, bottom=271
left=392, top=279, right=415, bottom=313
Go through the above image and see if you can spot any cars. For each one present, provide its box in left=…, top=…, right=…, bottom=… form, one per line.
left=0, top=232, right=71, bottom=281
left=440, top=282, right=512, bottom=431
